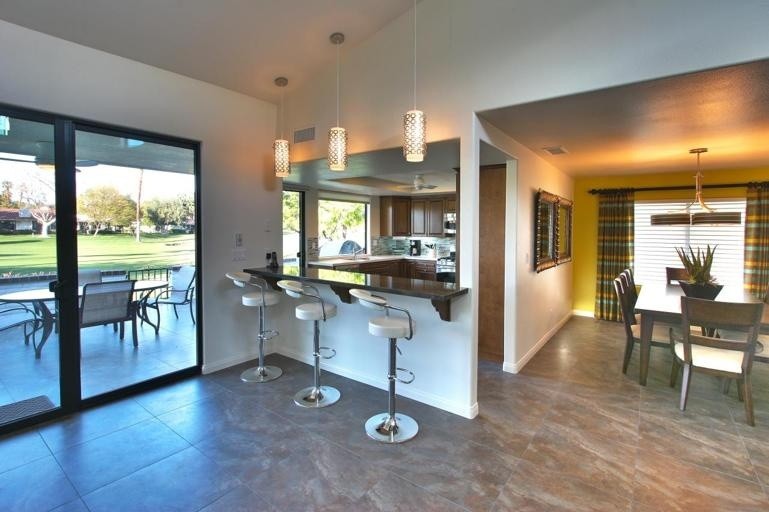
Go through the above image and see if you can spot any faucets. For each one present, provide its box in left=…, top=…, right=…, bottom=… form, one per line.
left=352, top=247, right=366, bottom=256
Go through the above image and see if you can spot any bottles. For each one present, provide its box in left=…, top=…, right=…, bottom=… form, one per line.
left=270, top=251, right=277, bottom=270
left=265, top=252, right=271, bottom=268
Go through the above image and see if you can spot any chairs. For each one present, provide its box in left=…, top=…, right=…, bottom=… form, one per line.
left=225, top=271, right=284, bottom=384
left=613, top=266, right=769, bottom=426
left=348, top=288, right=419, bottom=444
left=276, top=279, right=341, bottom=409
left=0, top=266, right=196, bottom=359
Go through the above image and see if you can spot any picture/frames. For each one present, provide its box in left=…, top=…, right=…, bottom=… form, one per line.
left=534, top=188, right=557, bottom=273
left=557, top=197, right=573, bottom=265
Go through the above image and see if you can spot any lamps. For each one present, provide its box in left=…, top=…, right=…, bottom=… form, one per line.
left=274, top=77, right=290, bottom=177
left=404, top=0, right=426, bottom=162
left=667, top=148, right=717, bottom=212
left=329, top=32, right=347, bottom=171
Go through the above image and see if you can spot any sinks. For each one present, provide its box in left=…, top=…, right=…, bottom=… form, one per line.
left=343, top=257, right=370, bottom=261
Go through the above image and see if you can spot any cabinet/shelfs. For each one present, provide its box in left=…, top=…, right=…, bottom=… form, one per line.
left=380, top=196, right=457, bottom=236
left=359, top=259, right=436, bottom=281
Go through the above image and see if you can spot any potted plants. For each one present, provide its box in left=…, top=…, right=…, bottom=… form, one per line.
left=675, top=245, right=724, bottom=300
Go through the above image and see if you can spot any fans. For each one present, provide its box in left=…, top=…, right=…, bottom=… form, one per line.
left=399, top=175, right=438, bottom=192
